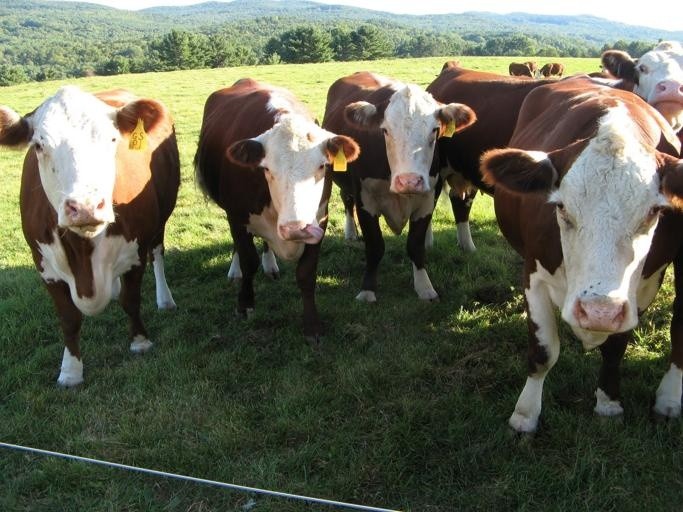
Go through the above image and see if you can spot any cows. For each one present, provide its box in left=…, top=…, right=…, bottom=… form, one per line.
left=425, top=39, right=683, bottom=452
left=321, top=70, right=478, bottom=305
left=192, top=77, right=362, bottom=348
left=0, top=83, right=181, bottom=388
